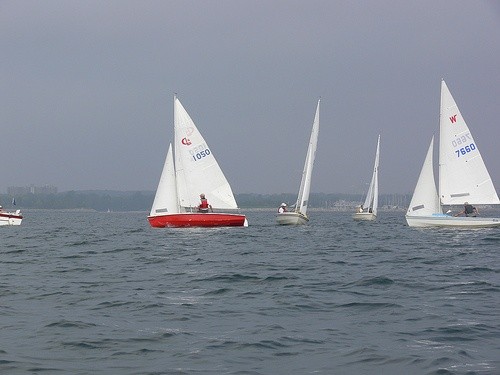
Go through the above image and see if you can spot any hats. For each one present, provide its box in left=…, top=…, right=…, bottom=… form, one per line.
left=281, top=203, right=286, bottom=206
left=200, top=194, right=204, bottom=198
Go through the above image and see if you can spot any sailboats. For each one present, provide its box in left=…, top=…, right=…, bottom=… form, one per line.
left=350, top=131, right=382, bottom=222
left=272, top=95, right=321, bottom=226
left=145, top=92, right=249, bottom=230
left=403, top=77, right=500, bottom=230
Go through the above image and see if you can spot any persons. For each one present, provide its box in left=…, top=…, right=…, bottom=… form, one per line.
left=197, top=193, right=213, bottom=213
left=359, top=205, right=364, bottom=213
left=278, top=203, right=289, bottom=214
left=455, top=202, right=481, bottom=217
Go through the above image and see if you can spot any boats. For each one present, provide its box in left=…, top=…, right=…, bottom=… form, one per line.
left=0, top=207, right=23, bottom=226
